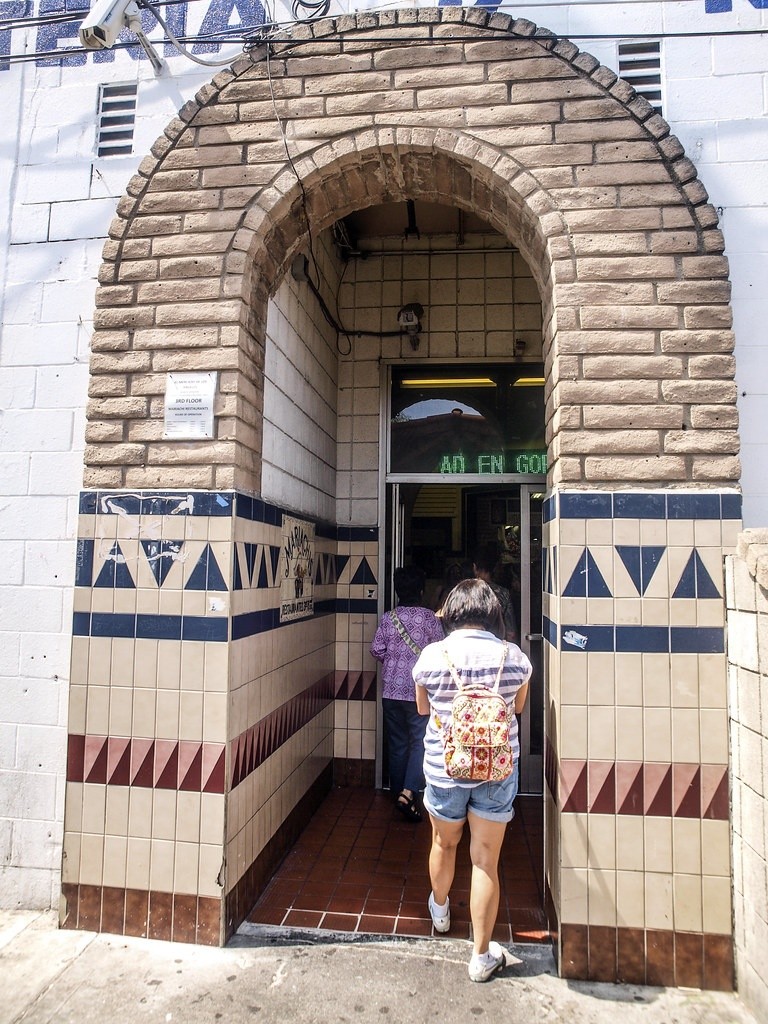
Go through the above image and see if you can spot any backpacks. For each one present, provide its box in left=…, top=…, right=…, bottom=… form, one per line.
left=437, top=639, right=514, bottom=782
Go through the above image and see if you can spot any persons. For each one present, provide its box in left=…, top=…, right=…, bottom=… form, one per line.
left=445, top=550, right=468, bottom=588
left=369, top=568, right=439, bottom=823
left=411, top=578, right=531, bottom=985
left=470, top=546, right=518, bottom=644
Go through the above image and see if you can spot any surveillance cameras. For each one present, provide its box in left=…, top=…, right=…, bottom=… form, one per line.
left=397, top=303, right=423, bottom=329
left=79, top=0, right=142, bottom=50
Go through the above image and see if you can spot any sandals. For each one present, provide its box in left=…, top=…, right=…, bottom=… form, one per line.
left=395, top=792, right=422, bottom=821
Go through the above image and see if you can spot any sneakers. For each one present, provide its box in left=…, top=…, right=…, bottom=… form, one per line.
left=428, top=890, right=452, bottom=933
left=468, top=941, right=504, bottom=982
